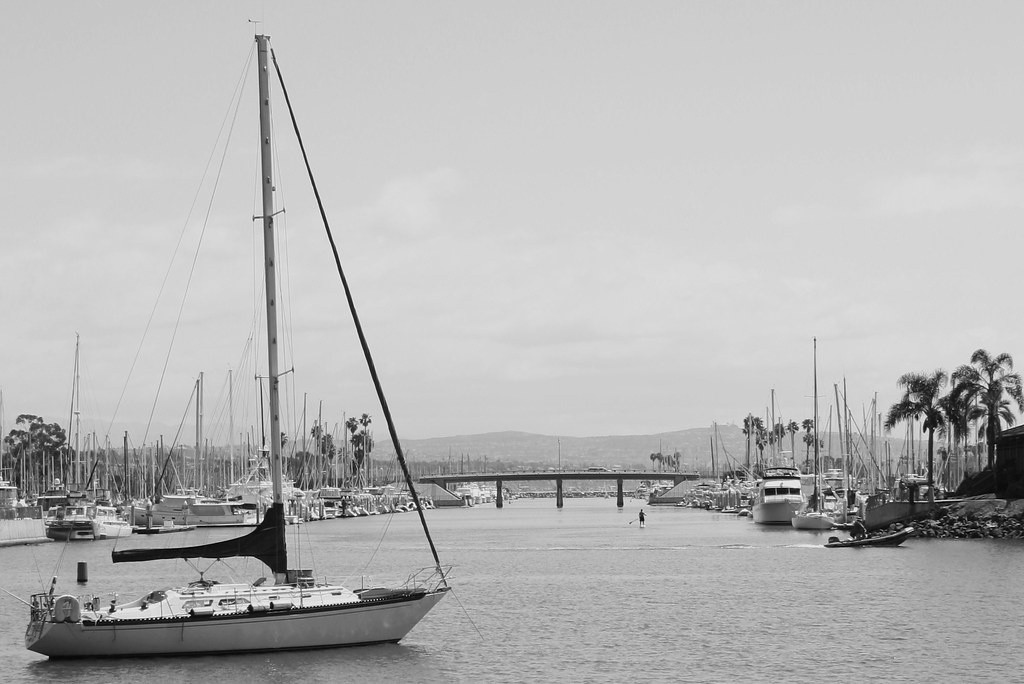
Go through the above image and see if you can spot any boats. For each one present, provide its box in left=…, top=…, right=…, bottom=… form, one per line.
left=824, top=527, right=915, bottom=547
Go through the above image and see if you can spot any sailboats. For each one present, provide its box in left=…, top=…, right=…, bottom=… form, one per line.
left=683, top=336, right=947, bottom=530
left=0, top=326, right=439, bottom=549
left=21, top=18, right=462, bottom=659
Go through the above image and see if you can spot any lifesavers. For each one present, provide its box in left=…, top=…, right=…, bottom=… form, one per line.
left=54, top=595, right=81, bottom=622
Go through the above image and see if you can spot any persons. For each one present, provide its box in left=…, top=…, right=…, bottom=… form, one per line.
left=912, top=481, right=920, bottom=500
left=854, top=517, right=866, bottom=539
left=638, top=509, right=647, bottom=528
left=342, top=499, right=346, bottom=514
